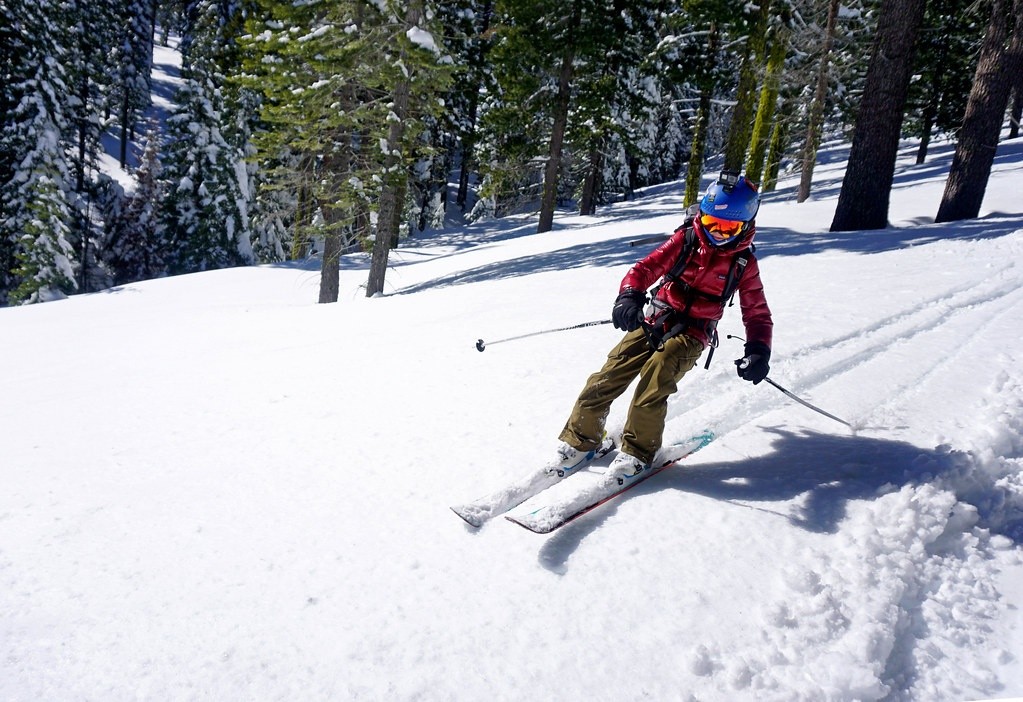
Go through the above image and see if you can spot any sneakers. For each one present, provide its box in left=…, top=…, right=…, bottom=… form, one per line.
left=546, top=440, right=603, bottom=475
left=607, top=446, right=665, bottom=479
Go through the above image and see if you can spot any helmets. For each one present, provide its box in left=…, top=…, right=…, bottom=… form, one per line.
left=699, top=170, right=762, bottom=222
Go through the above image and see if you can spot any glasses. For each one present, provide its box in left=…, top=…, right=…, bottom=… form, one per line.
left=699, top=214, right=745, bottom=240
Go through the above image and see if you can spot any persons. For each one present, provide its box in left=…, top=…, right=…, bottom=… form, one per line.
left=552, top=169, right=773, bottom=476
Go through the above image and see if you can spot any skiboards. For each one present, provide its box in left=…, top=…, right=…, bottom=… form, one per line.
left=448, top=428, right=713, bottom=533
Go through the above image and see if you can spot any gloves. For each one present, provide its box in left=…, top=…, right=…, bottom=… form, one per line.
left=612, top=289, right=648, bottom=332
left=734, top=340, right=771, bottom=385
